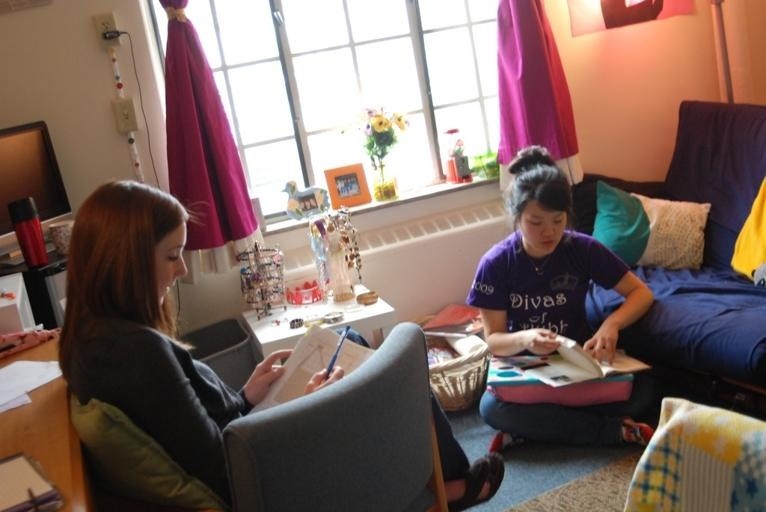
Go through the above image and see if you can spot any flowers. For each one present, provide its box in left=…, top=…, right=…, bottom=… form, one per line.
left=363, top=107, right=406, bottom=200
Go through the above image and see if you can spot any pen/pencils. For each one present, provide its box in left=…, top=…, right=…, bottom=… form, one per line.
left=328, top=325, right=351, bottom=374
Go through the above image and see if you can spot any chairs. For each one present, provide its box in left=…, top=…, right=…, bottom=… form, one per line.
left=220, top=322, right=451, bottom=512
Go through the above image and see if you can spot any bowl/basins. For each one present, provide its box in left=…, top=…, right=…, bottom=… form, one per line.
left=285, top=274, right=332, bottom=305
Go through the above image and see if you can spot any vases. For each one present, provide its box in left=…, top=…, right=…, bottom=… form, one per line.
left=373, top=176, right=399, bottom=203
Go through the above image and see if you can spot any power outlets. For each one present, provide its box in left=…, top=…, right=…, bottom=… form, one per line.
left=91, top=12, right=120, bottom=46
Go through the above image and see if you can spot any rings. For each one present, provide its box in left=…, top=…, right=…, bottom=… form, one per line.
left=530, top=342, right=538, bottom=349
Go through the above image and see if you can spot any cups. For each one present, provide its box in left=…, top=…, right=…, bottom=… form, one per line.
left=47, top=219, right=76, bottom=255
left=8, top=196, right=47, bottom=269
left=312, top=233, right=356, bottom=303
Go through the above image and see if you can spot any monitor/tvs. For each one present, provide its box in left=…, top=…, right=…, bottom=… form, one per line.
left=0, top=120, right=72, bottom=265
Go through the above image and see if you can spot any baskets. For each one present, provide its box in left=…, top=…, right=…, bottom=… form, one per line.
left=423, top=334, right=489, bottom=412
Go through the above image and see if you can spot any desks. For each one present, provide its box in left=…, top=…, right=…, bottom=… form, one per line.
left=241, top=283, right=396, bottom=366
left=0, top=325, right=100, bottom=510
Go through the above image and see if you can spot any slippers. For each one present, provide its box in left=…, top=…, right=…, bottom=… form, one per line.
left=446, top=450, right=507, bottom=512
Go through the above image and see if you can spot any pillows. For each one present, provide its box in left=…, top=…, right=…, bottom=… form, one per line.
left=590, top=179, right=713, bottom=270
left=69, top=392, right=231, bottom=512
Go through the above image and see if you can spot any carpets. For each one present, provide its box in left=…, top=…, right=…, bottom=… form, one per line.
left=503, top=449, right=644, bottom=511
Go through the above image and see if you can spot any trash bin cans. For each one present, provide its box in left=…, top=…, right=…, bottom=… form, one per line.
left=180, top=317, right=258, bottom=392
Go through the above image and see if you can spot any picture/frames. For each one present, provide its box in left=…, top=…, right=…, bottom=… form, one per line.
left=324, top=162, right=372, bottom=210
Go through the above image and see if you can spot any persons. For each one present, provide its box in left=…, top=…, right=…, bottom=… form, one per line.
left=335, top=175, right=361, bottom=198
left=464, top=144, right=659, bottom=456
left=298, top=196, right=317, bottom=212
left=56, top=177, right=506, bottom=512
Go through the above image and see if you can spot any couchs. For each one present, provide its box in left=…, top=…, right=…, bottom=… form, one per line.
left=571, top=99, right=766, bottom=397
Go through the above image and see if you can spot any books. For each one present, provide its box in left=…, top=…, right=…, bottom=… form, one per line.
left=510, top=330, right=655, bottom=391
left=241, top=321, right=379, bottom=417
